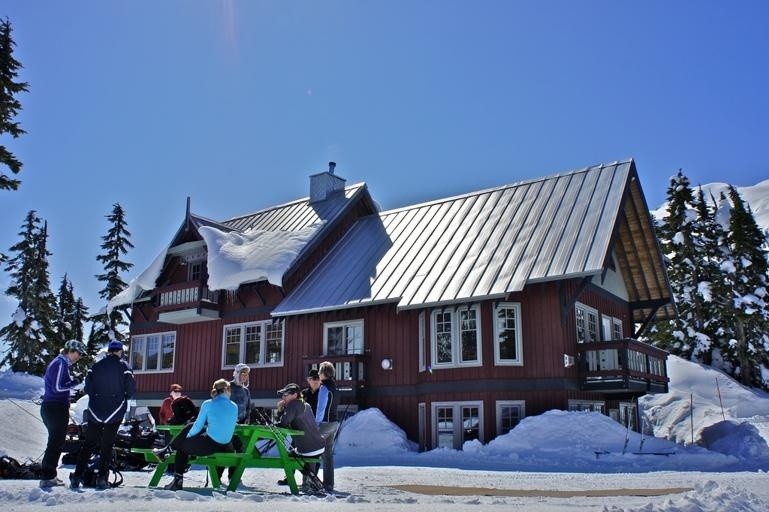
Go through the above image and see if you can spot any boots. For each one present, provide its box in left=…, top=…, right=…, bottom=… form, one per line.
left=152, top=446, right=173, bottom=462
left=164, top=475, right=184, bottom=491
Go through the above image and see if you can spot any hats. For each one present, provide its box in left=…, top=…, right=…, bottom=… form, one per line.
left=278, top=383, right=299, bottom=394
left=234, top=364, right=249, bottom=386
left=109, top=340, right=124, bottom=357
left=66, top=340, right=87, bottom=355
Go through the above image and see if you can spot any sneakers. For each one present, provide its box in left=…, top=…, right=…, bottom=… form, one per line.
left=279, top=479, right=287, bottom=484
left=97, top=477, right=110, bottom=488
left=70, top=474, right=80, bottom=487
left=40, top=477, right=65, bottom=488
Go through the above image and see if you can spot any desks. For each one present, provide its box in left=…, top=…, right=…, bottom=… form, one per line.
left=131, top=425, right=321, bottom=495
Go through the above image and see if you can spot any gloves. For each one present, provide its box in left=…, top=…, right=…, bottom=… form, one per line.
left=75, top=373, right=84, bottom=383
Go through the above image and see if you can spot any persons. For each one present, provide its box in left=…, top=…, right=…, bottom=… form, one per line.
left=216, top=363, right=252, bottom=485
left=69, top=340, right=136, bottom=491
left=38, top=340, right=88, bottom=488
left=152, top=379, right=239, bottom=491
left=300, top=369, right=321, bottom=488
left=158, top=383, right=183, bottom=472
left=273, top=382, right=326, bottom=485
left=298, top=361, right=339, bottom=493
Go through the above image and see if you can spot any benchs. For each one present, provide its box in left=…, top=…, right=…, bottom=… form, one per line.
left=132, top=447, right=247, bottom=465
left=253, top=456, right=321, bottom=469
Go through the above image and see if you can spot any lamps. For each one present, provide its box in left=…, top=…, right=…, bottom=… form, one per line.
left=381, top=359, right=393, bottom=370
